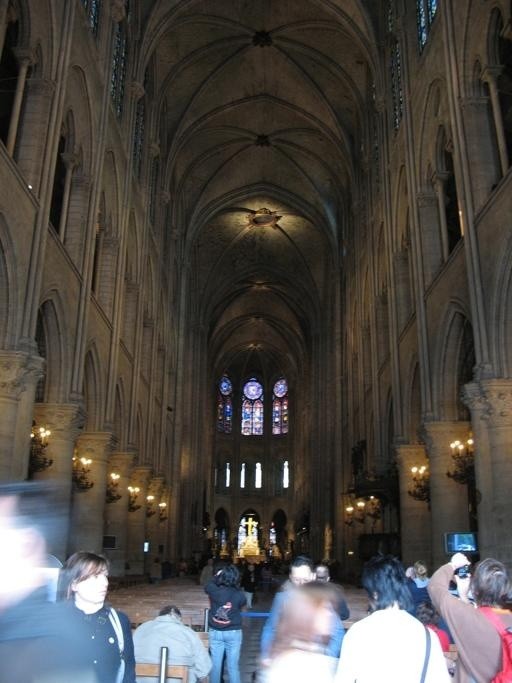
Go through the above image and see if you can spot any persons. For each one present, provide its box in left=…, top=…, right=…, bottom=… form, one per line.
left=48, top=548, right=137, bottom=682
left=262, top=555, right=511, bottom=683
left=132, top=547, right=261, bottom=681
left=0, top=484, right=105, bottom=681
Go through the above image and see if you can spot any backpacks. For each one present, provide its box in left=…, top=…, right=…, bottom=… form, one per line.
left=479, top=606, right=512, bottom=682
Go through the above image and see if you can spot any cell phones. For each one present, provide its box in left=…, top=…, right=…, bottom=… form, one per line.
left=458, top=564, right=470, bottom=578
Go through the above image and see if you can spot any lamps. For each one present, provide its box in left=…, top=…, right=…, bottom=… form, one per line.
left=28, top=425, right=140, bottom=514
left=405, top=435, right=474, bottom=502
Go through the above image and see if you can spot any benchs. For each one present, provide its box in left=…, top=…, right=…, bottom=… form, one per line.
left=106, top=578, right=210, bottom=682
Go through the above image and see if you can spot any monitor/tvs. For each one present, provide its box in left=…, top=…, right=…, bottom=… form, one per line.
left=143, top=540, right=151, bottom=553
left=443, top=530, right=479, bottom=554
left=159, top=544, right=164, bottom=554
left=103, top=534, right=118, bottom=549
